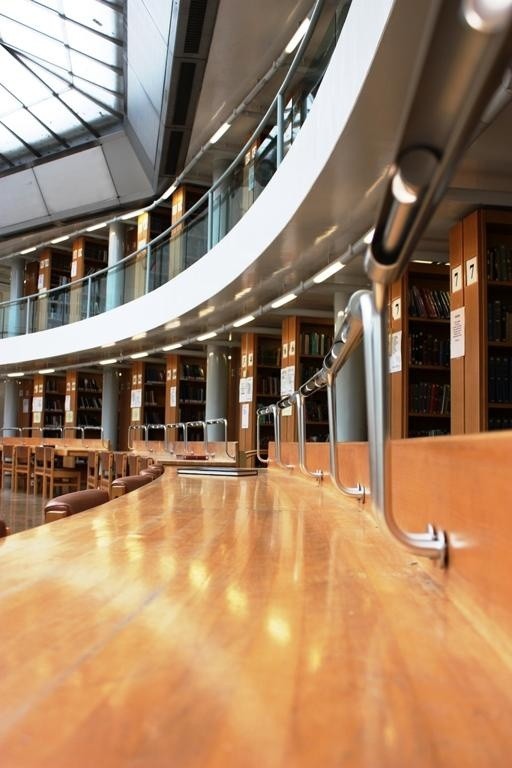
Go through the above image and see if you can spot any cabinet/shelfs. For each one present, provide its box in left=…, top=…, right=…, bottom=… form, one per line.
left=18, top=73, right=512, bottom=469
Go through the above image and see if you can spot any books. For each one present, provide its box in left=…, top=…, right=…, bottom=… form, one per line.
left=406, top=280, right=455, bottom=438
left=142, top=366, right=167, bottom=441
left=174, top=363, right=205, bottom=442
left=49, top=252, right=70, bottom=327
left=76, top=375, right=103, bottom=439
left=487, top=223, right=510, bottom=433
left=80, top=241, right=108, bottom=320
left=224, top=170, right=274, bottom=237
left=255, top=344, right=282, bottom=442
left=176, top=464, right=259, bottom=476
left=148, top=210, right=174, bottom=293
left=183, top=169, right=243, bottom=270
left=297, top=329, right=333, bottom=442
left=41, top=378, right=65, bottom=439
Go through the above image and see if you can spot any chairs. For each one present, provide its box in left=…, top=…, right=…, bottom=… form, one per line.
left=1, top=444, right=165, bottom=523
